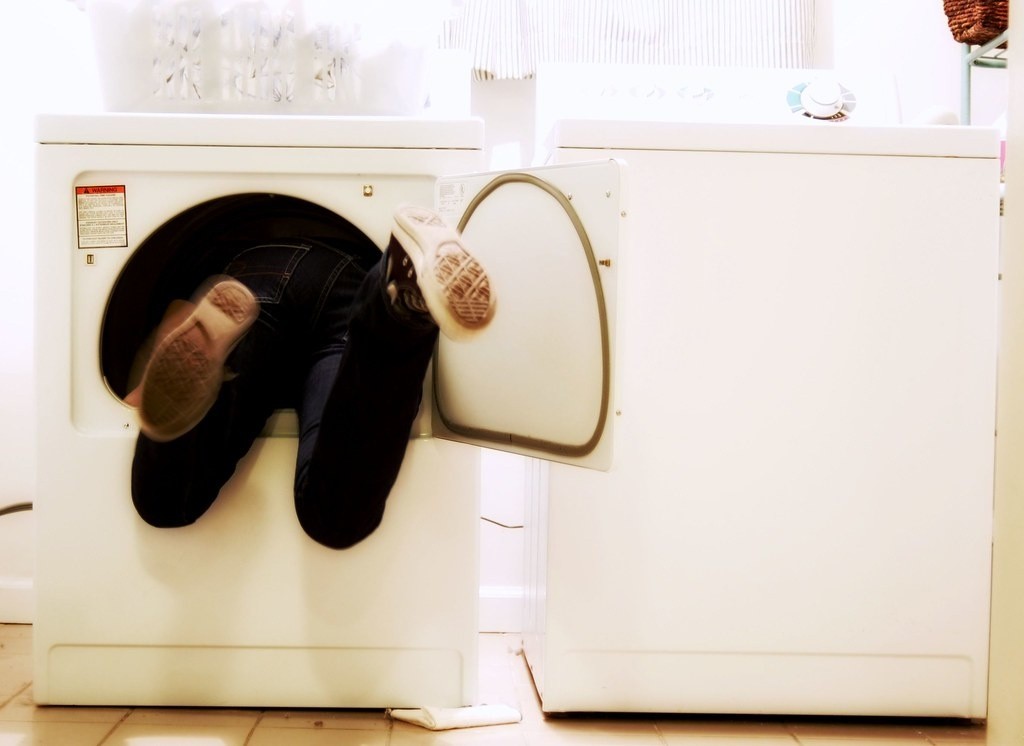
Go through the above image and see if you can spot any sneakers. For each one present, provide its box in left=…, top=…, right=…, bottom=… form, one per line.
left=138, top=280, right=258, bottom=441
left=391, top=202, right=497, bottom=342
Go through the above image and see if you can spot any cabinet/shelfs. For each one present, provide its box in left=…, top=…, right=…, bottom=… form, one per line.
left=960, top=27, right=1011, bottom=281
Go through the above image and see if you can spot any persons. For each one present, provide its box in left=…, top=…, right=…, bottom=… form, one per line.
left=117, top=207, right=498, bottom=551
